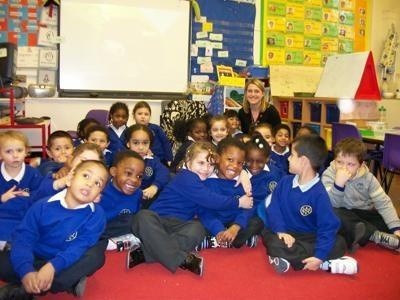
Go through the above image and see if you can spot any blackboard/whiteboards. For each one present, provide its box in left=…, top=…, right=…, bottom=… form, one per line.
left=57, top=0, right=193, bottom=99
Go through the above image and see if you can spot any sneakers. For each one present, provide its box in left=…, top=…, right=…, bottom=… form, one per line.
left=125, top=247, right=146, bottom=270
left=209, top=235, right=243, bottom=249
left=181, top=252, right=204, bottom=277
left=268, top=255, right=290, bottom=274
left=325, top=255, right=358, bottom=275
left=1, top=284, right=35, bottom=300
left=63, top=276, right=86, bottom=298
left=370, top=230, right=399, bottom=250
left=244, top=233, right=258, bottom=249
left=106, top=233, right=143, bottom=252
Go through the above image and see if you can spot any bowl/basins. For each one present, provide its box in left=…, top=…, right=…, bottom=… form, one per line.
left=382, top=89, right=395, bottom=99
left=294, top=91, right=315, bottom=97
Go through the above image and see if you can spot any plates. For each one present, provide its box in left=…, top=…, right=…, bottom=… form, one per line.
left=14, top=117, right=44, bottom=125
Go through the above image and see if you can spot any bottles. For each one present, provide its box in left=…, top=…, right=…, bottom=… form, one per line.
left=378, top=106, right=387, bottom=130
left=395, top=88, right=400, bottom=99
left=381, top=77, right=390, bottom=93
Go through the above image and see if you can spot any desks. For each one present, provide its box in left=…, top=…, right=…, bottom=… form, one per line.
left=360, top=126, right=400, bottom=195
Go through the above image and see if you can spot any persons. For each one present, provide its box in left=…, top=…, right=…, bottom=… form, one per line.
left=125, top=140, right=254, bottom=276
left=1, top=100, right=400, bottom=253
left=0, top=159, right=109, bottom=300
left=238, top=79, right=280, bottom=133
left=260, top=134, right=361, bottom=276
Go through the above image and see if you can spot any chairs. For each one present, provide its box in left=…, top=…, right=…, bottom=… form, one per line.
left=381, top=133, right=400, bottom=195
left=66, top=110, right=110, bottom=139
left=332, top=122, right=384, bottom=185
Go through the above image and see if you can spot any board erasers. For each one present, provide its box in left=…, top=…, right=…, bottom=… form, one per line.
left=90, top=93, right=98, bottom=97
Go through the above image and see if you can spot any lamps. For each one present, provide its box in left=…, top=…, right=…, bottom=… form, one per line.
left=335, top=97, right=355, bottom=115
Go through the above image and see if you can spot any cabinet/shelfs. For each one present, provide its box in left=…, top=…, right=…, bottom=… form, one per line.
left=271, top=95, right=379, bottom=153
left=0, top=89, right=51, bottom=165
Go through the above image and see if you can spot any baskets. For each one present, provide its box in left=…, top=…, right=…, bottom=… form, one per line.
left=305, top=124, right=320, bottom=138
left=279, top=100, right=289, bottom=118
left=247, top=65, right=269, bottom=78
left=293, top=102, right=340, bottom=124
